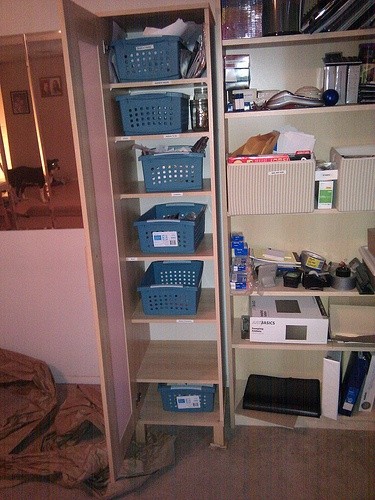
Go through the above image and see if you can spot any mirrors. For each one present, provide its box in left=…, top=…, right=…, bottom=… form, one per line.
left=0, top=30, right=83, bottom=232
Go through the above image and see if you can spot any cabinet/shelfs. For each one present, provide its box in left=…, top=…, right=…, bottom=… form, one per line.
left=214, top=0, right=375, bottom=432
left=59, top=0, right=227, bottom=482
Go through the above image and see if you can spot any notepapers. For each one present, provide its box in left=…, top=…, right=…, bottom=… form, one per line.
left=263, top=248, right=286, bottom=260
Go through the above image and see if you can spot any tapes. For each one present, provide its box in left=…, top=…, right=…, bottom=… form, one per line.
left=309, top=270, right=319, bottom=277
left=300, top=250, right=325, bottom=271
left=330, top=270, right=356, bottom=290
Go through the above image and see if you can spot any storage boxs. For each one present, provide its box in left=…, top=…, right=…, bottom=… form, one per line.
left=230, top=232, right=248, bottom=289
left=223, top=55, right=280, bottom=112
left=133, top=202, right=208, bottom=253
left=110, top=35, right=188, bottom=82
left=249, top=295, right=329, bottom=345
left=139, top=144, right=205, bottom=193
left=114, top=92, right=190, bottom=136
left=228, top=147, right=375, bottom=216
left=157, top=383, right=215, bottom=412
left=328, top=296, right=375, bottom=343
left=137, top=260, right=204, bottom=315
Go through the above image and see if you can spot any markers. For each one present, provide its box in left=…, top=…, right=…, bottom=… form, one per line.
left=325, top=261, right=332, bottom=270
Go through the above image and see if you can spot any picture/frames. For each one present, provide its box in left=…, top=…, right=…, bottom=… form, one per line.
left=39, top=76, right=62, bottom=98
left=10, top=90, right=30, bottom=115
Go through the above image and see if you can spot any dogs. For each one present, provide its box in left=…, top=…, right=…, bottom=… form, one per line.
left=2, top=158, right=60, bottom=204
